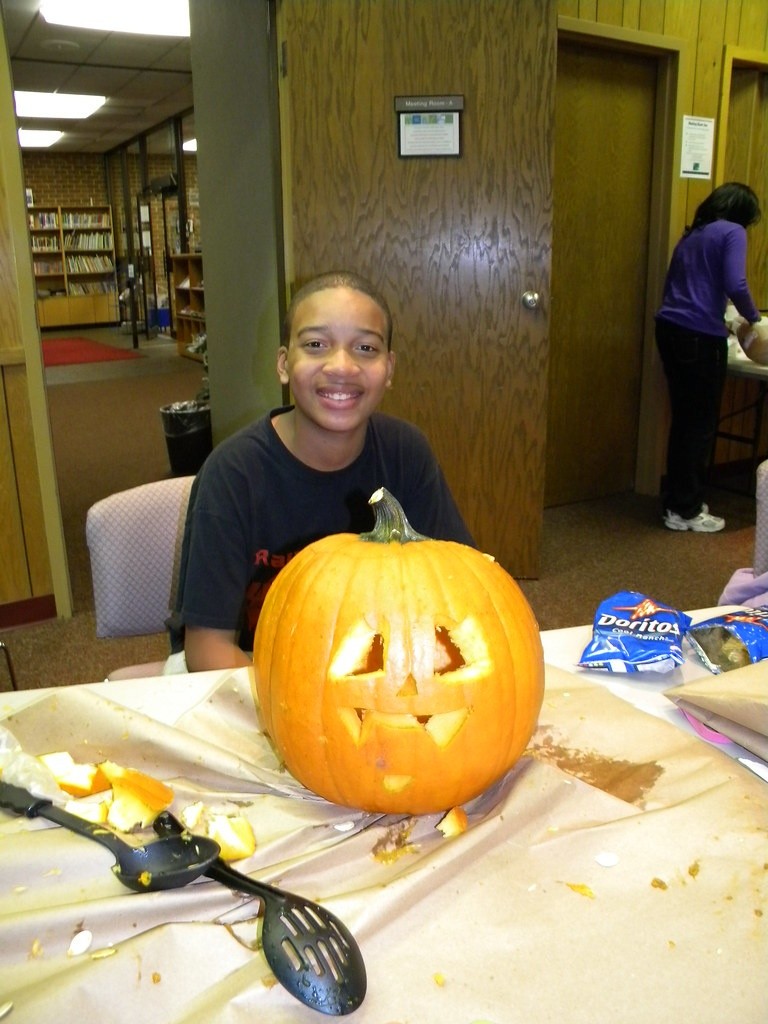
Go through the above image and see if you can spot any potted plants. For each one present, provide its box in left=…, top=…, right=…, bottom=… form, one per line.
left=159, top=332, right=212, bottom=478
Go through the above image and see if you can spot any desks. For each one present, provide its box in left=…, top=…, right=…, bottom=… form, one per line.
left=718, top=359, right=768, bottom=497
left=0, top=603, right=768, bottom=1024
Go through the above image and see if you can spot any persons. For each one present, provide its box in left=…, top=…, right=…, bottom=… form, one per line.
left=163, top=271, right=474, bottom=682
left=655, top=182, right=762, bottom=534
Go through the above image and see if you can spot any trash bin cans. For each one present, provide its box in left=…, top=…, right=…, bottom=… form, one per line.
left=158, top=399, right=213, bottom=477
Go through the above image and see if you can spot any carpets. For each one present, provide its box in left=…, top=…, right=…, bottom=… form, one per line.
left=42, top=336, right=149, bottom=368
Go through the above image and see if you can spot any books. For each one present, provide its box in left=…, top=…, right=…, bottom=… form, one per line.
left=34, top=254, right=113, bottom=273
left=27, top=213, right=111, bottom=229
left=68, top=280, right=116, bottom=296
left=31, top=232, right=113, bottom=252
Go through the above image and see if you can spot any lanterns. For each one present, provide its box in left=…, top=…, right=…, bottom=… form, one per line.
left=252, top=533, right=545, bottom=814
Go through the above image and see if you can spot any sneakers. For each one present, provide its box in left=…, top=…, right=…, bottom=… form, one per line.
left=662, top=503, right=725, bottom=533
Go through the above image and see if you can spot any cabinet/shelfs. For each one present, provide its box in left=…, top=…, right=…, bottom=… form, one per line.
left=27, top=204, right=121, bottom=327
left=168, top=252, right=206, bottom=361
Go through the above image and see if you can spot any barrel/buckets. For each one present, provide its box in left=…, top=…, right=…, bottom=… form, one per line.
left=148, top=308, right=170, bottom=327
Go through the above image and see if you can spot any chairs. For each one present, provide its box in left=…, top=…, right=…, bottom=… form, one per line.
left=87, top=475, right=196, bottom=683
